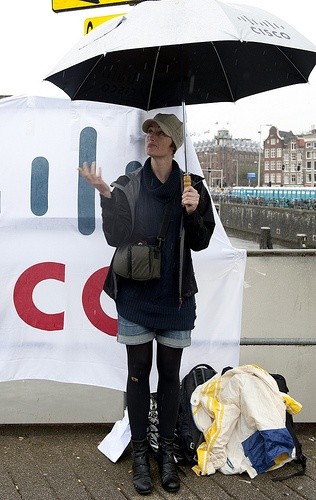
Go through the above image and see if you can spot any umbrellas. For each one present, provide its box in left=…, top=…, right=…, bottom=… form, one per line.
left=42, top=0, right=316, bottom=206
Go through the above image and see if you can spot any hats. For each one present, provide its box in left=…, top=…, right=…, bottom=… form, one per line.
left=142, top=113, right=184, bottom=150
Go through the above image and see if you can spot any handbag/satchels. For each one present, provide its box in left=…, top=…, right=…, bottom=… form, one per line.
left=112, top=240, right=161, bottom=281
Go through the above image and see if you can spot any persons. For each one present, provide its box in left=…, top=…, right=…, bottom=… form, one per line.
left=77, top=113, right=215, bottom=495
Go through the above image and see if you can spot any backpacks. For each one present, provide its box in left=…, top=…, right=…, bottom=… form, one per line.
left=221, top=365, right=301, bottom=464
left=177, top=363, right=218, bottom=464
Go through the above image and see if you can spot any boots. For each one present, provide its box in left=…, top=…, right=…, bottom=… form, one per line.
left=157, top=436, right=180, bottom=491
left=130, top=436, right=154, bottom=493
